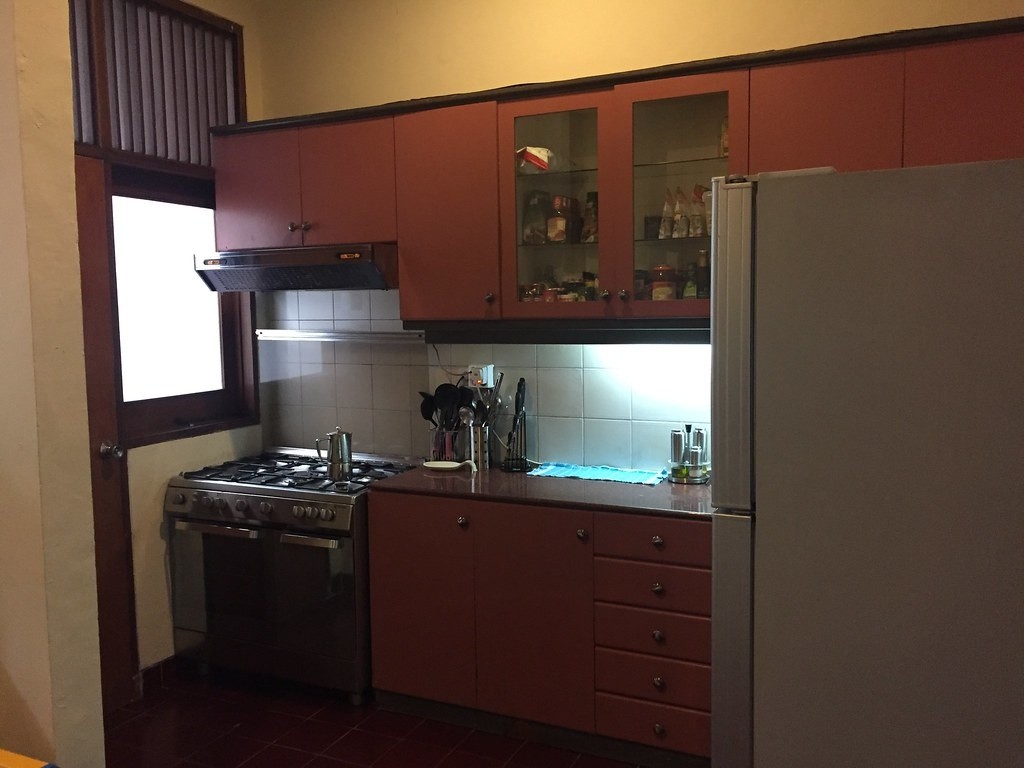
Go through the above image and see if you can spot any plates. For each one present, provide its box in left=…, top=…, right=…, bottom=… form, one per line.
left=423, top=460, right=461, bottom=470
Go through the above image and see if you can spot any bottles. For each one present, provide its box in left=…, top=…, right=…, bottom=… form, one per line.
left=518, top=187, right=603, bottom=303
left=670, top=426, right=708, bottom=467
left=632, top=249, right=711, bottom=301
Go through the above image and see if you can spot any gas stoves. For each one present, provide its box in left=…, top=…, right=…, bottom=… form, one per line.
left=180, top=451, right=416, bottom=497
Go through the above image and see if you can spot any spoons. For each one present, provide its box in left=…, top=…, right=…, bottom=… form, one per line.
left=475, top=400, right=489, bottom=427
left=459, top=406, right=474, bottom=426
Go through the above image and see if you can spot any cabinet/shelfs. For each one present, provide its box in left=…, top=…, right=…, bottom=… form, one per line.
left=747, top=31, right=1024, bottom=176
left=363, top=489, right=477, bottom=709
left=499, top=69, right=751, bottom=321
left=211, top=114, right=398, bottom=251
left=395, top=100, right=502, bottom=322
left=476, top=498, right=594, bottom=736
left=593, top=510, right=712, bottom=761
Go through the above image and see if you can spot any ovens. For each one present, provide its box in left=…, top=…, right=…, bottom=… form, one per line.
left=162, top=486, right=368, bottom=709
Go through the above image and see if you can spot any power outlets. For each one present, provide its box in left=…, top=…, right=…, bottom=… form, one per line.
left=467, top=363, right=495, bottom=389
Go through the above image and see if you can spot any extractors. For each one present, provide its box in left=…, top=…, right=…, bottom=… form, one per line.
left=192, top=245, right=399, bottom=293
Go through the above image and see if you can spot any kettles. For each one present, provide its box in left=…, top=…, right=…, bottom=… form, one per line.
left=315, top=424, right=354, bottom=482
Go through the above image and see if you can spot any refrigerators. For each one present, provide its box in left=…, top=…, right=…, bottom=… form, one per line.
left=710, top=155, right=1024, bottom=768
